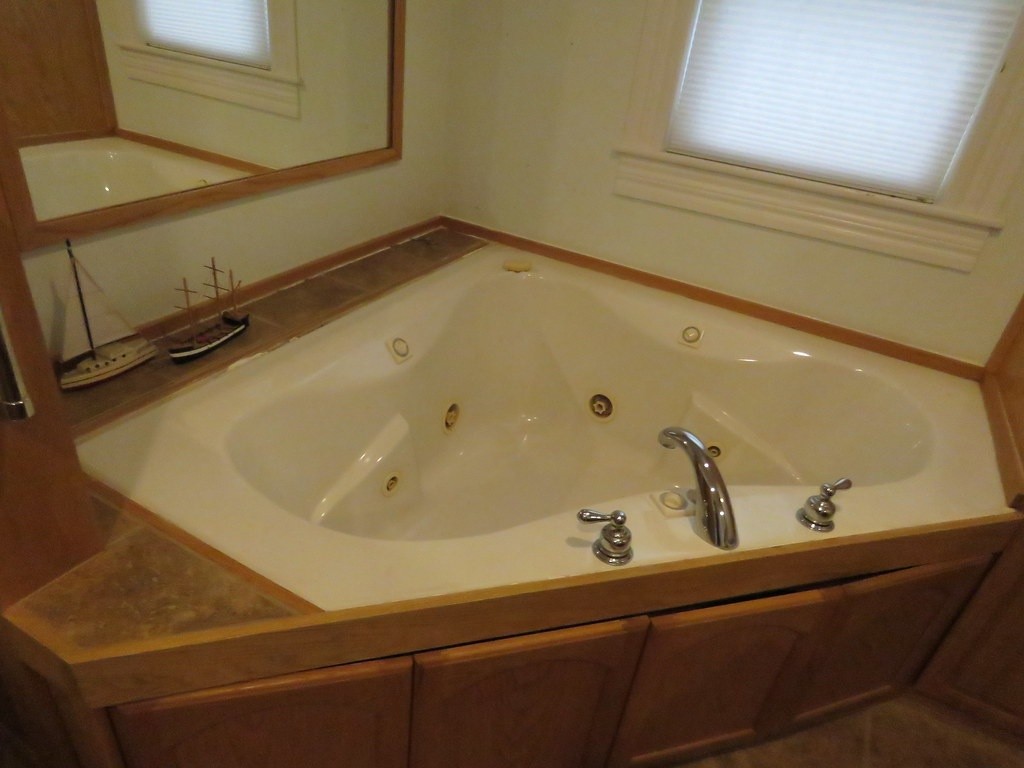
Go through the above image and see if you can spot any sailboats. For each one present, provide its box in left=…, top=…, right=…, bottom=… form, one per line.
left=164, top=256, right=251, bottom=364
left=55, top=237, right=161, bottom=393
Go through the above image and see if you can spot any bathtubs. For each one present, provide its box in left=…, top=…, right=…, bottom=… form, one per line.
left=10, top=126, right=279, bottom=221
left=74, top=237, right=1024, bottom=768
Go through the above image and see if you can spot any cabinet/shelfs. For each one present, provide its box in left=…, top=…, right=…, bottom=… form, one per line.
left=0, top=524, right=1024, bottom=768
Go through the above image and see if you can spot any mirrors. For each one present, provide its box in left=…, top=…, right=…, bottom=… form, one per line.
left=0, top=0, right=408, bottom=251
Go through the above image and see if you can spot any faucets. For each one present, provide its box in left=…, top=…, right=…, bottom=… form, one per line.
left=658, top=427, right=740, bottom=549
